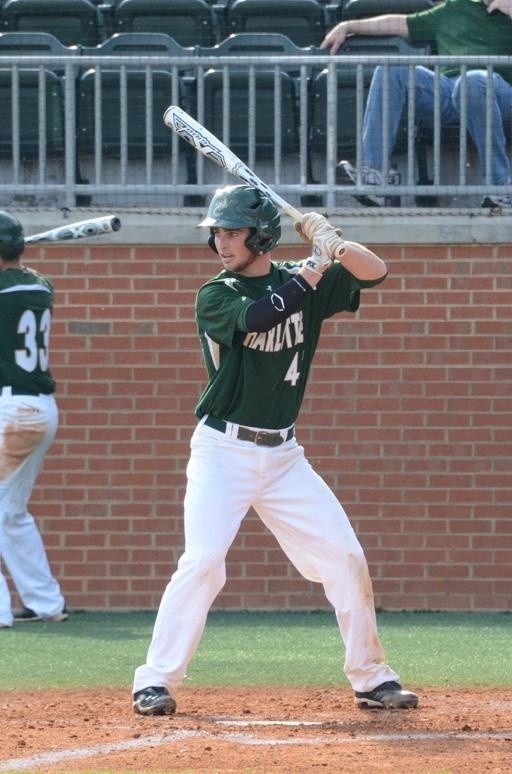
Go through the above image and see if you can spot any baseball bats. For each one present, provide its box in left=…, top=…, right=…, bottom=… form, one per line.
left=24, top=215, right=120, bottom=245
left=162, top=106, right=346, bottom=258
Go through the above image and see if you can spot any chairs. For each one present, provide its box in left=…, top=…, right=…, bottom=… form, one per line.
left=107, top=1, right=218, bottom=48
left=324, top=3, right=439, bottom=59
left=419, top=25, right=510, bottom=211
left=206, top=0, right=327, bottom=48
left=193, top=23, right=317, bottom=209
left=3, top=2, right=104, bottom=48
left=0, top=31, right=74, bottom=209
left=72, top=29, right=193, bottom=207
left=308, top=19, right=421, bottom=208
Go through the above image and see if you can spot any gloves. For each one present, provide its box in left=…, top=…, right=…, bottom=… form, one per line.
left=293, top=211, right=335, bottom=241
left=302, top=224, right=344, bottom=277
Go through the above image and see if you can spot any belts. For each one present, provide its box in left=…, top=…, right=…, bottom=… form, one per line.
left=203, top=416, right=295, bottom=447
left=0, top=386, right=40, bottom=397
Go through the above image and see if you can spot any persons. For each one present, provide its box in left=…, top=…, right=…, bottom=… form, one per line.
left=131, top=183, right=419, bottom=715
left=0, top=209, right=70, bottom=626
left=318, top=0, right=512, bottom=207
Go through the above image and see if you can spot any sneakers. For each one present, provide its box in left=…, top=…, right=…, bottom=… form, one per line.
left=336, top=160, right=402, bottom=207
left=354, top=680, right=419, bottom=709
left=133, top=686, right=177, bottom=716
left=10, top=607, right=69, bottom=622
left=481, top=194, right=512, bottom=209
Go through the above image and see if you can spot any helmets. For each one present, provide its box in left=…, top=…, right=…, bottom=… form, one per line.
left=194, top=184, right=282, bottom=256
left=0, top=211, right=25, bottom=257
